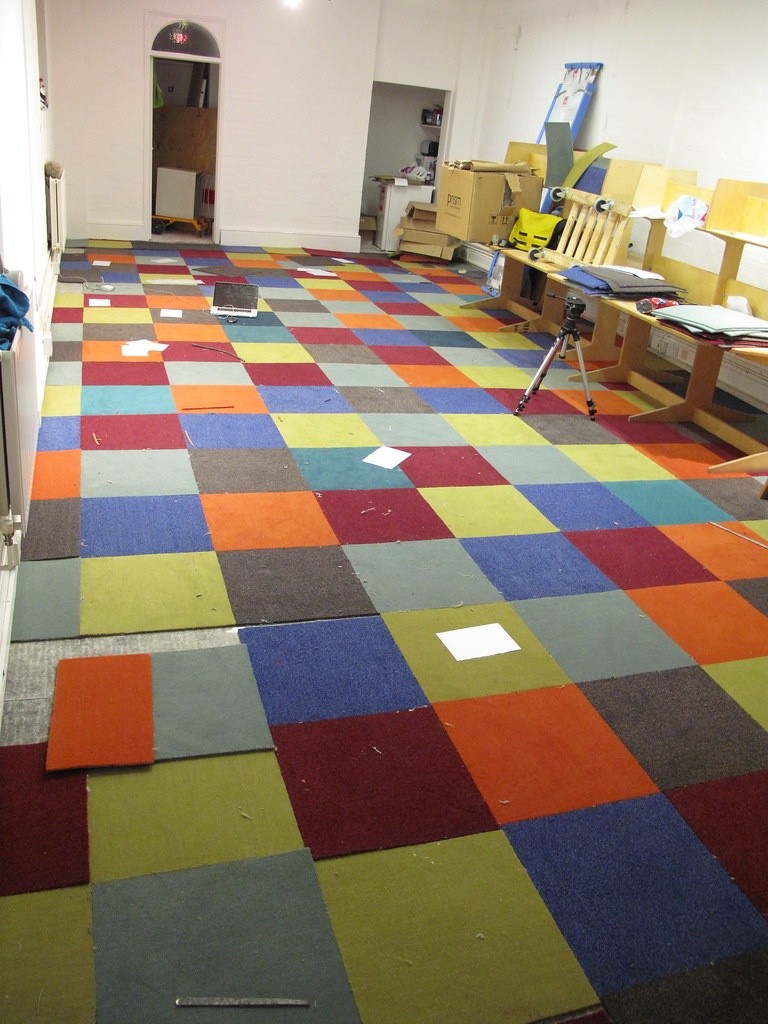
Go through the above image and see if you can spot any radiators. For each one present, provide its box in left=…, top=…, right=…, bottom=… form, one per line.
left=49, top=170, right=65, bottom=253
left=0, top=285, right=40, bottom=537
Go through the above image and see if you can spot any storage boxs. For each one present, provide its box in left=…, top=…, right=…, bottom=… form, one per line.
left=398, top=236, right=462, bottom=261
left=391, top=224, right=458, bottom=246
left=358, top=212, right=376, bottom=246
left=405, top=201, right=437, bottom=221
left=435, top=164, right=543, bottom=243
left=400, top=216, right=447, bottom=234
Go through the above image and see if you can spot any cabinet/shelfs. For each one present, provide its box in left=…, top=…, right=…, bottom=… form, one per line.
left=375, top=182, right=436, bottom=252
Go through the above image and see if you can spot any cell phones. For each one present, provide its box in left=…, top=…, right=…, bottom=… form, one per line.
left=227, top=318, right=238, bottom=323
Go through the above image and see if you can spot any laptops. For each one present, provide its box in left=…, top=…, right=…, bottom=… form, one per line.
left=210, top=282, right=258, bottom=317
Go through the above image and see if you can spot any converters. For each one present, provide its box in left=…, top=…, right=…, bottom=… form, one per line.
left=101, top=285, right=114, bottom=292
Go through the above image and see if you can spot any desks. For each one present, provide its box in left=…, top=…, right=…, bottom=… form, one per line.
left=458, top=141, right=768, bottom=499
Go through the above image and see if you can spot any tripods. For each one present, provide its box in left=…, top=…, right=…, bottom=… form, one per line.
left=513, top=293, right=596, bottom=422
